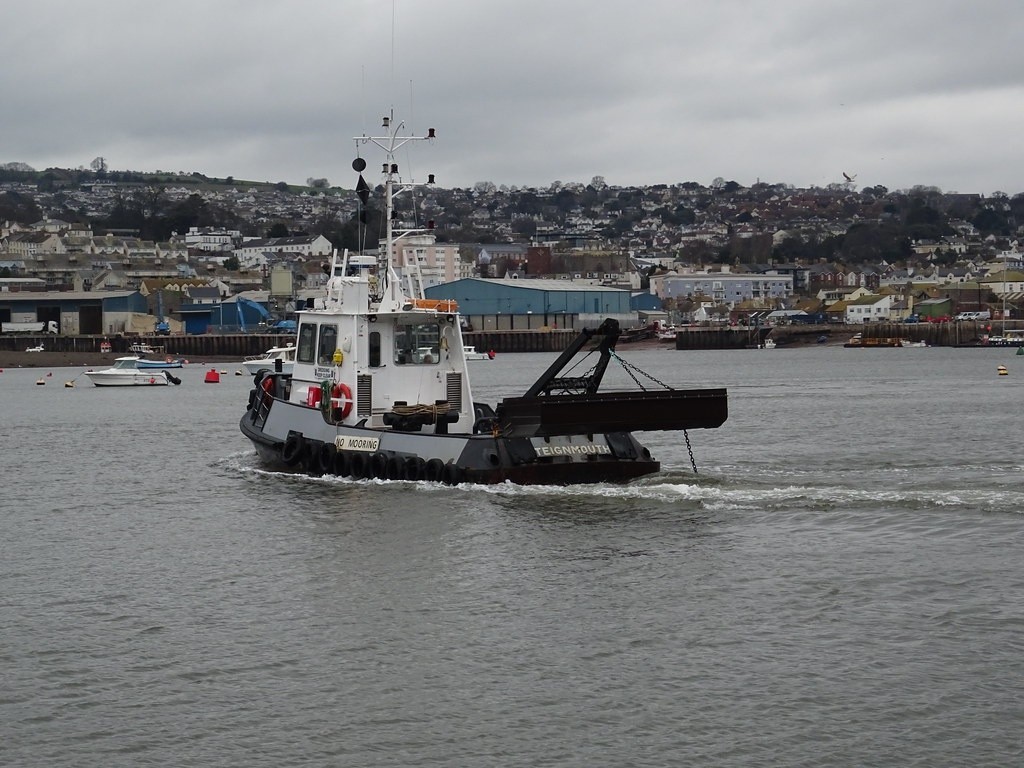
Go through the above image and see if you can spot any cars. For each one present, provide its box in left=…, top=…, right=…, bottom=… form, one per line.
left=904, top=313, right=927, bottom=323
left=932, top=316, right=954, bottom=323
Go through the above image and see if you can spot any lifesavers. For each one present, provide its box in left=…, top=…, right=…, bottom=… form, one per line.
left=281, top=436, right=462, bottom=488
left=332, top=386, right=351, bottom=419
left=167, top=356, right=173, bottom=363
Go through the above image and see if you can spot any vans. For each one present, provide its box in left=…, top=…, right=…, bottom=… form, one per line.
left=954, top=311, right=977, bottom=320
left=974, top=311, right=991, bottom=321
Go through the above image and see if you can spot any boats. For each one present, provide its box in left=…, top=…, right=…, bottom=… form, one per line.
left=618, top=322, right=654, bottom=343
left=127, top=342, right=159, bottom=355
left=241, top=338, right=297, bottom=377
left=757, top=338, right=776, bottom=349
left=238, top=106, right=729, bottom=487
left=137, top=354, right=189, bottom=370
left=87, top=354, right=181, bottom=392
left=651, top=314, right=678, bottom=341
